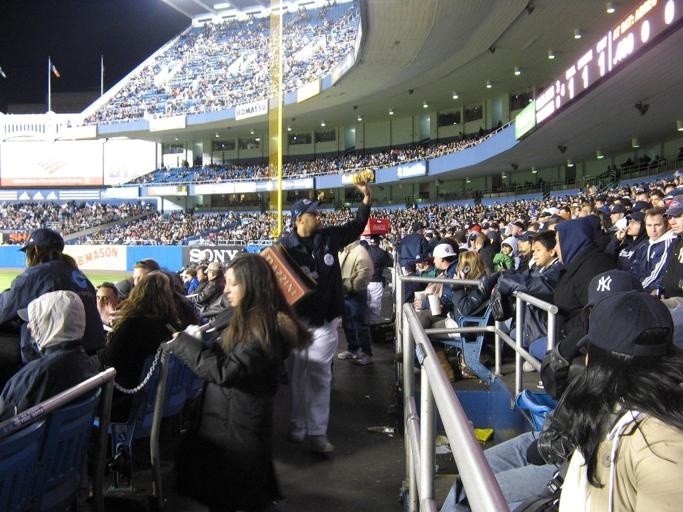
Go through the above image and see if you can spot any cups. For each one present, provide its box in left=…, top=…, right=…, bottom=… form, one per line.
left=413, top=291, right=426, bottom=312
left=427, top=293, right=441, bottom=316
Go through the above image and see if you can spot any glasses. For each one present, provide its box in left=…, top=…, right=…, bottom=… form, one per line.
left=96, top=295, right=111, bottom=303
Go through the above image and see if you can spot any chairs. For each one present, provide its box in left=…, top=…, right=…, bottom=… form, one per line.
left=0, top=334, right=212, bottom=512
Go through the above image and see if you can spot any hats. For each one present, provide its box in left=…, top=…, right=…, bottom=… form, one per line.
left=414, top=254, right=427, bottom=263
left=413, top=222, right=426, bottom=231
left=577, top=290, right=673, bottom=357
left=463, top=185, right=682, bottom=257
left=16, top=307, right=29, bottom=323
left=19, top=228, right=64, bottom=252
left=179, top=270, right=187, bottom=277
left=492, top=290, right=514, bottom=322
left=290, top=198, right=321, bottom=218
left=204, top=262, right=223, bottom=273
left=432, top=244, right=457, bottom=258
left=370, top=236, right=380, bottom=243
left=582, top=269, right=642, bottom=312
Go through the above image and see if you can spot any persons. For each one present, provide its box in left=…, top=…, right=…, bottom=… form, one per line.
left=86, top=0, right=359, bottom=123
left=0, top=119, right=393, bottom=512
left=380, top=122, right=683, bottom=511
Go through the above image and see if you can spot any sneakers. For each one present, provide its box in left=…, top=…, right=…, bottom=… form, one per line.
left=306, top=437, right=334, bottom=454
left=357, top=353, right=373, bottom=364
left=289, top=426, right=306, bottom=442
left=338, top=350, right=359, bottom=359
